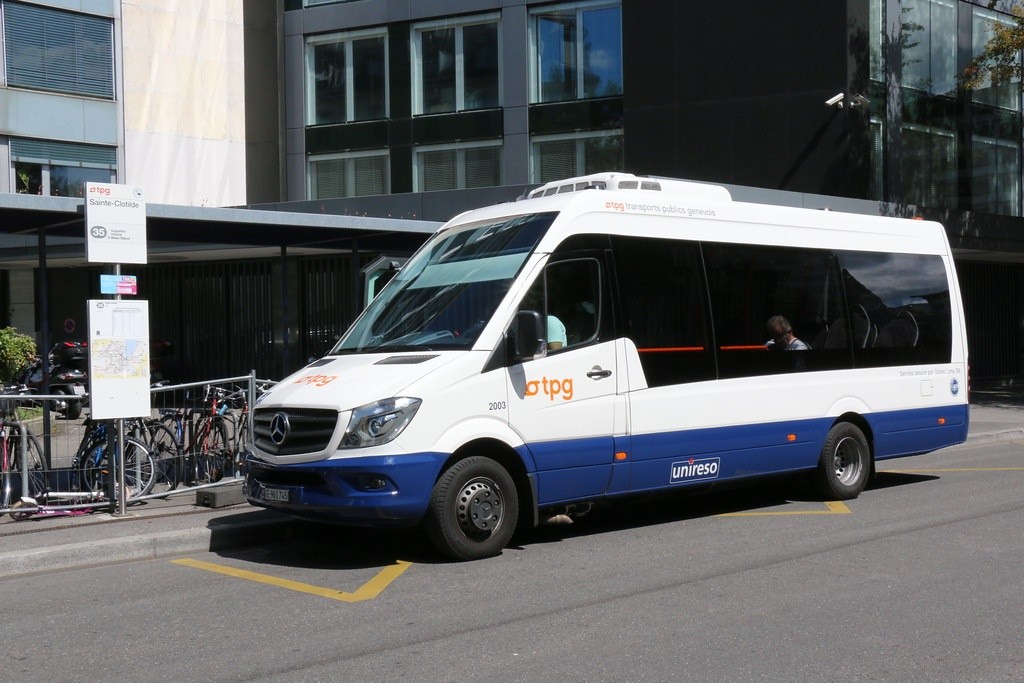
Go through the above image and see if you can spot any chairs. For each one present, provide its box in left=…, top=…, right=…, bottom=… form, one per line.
left=814, top=303, right=920, bottom=351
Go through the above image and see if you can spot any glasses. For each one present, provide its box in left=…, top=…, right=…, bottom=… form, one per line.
left=774, top=333, right=786, bottom=344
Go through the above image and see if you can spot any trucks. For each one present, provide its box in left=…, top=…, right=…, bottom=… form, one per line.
left=243, top=172, right=969, bottom=562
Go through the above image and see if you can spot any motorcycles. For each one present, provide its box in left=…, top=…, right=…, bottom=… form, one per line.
left=24, top=338, right=88, bottom=419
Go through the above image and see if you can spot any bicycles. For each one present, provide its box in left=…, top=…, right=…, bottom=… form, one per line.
left=0, top=379, right=270, bottom=517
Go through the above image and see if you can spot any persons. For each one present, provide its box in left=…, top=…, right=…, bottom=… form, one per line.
left=763, top=315, right=808, bottom=350
left=523, top=284, right=565, bottom=352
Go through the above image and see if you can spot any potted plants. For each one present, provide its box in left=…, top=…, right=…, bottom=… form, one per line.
left=0, top=326, right=37, bottom=415
